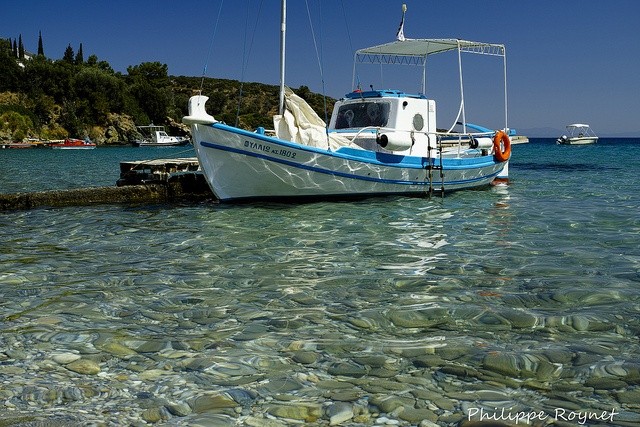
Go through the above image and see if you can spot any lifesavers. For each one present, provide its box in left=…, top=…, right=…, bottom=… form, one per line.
left=494, top=131, right=510, bottom=161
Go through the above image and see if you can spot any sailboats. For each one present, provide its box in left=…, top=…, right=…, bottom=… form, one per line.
left=182, top=0, right=516, bottom=205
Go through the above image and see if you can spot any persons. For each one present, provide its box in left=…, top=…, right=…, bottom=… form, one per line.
left=335, top=111, right=349, bottom=129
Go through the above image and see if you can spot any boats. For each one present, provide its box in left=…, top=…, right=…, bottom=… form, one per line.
left=47, top=136, right=97, bottom=150
left=0, top=143, right=37, bottom=148
left=134, top=130, right=183, bottom=146
left=555, top=123, right=599, bottom=146
